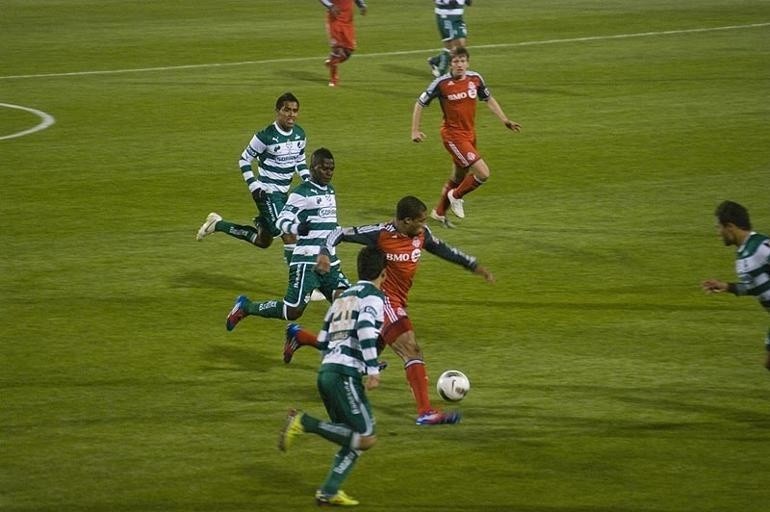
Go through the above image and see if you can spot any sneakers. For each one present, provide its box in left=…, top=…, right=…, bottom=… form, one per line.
left=447, top=189, right=465, bottom=219
left=197, top=212, right=223, bottom=241
left=314, top=488, right=360, bottom=507
left=225, top=294, right=250, bottom=332
left=426, top=57, right=440, bottom=78
left=281, top=321, right=302, bottom=363
left=278, top=408, right=305, bottom=453
left=432, top=69, right=445, bottom=77
left=430, top=207, right=456, bottom=229
left=323, top=58, right=336, bottom=67
left=415, top=408, right=461, bottom=427
left=329, top=75, right=338, bottom=86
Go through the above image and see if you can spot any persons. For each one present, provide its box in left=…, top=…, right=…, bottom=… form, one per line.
left=226, top=147, right=388, bottom=372
left=277, top=246, right=387, bottom=507
left=702, top=199, right=770, bottom=369
left=196, top=91, right=326, bottom=302
left=427, top=0, right=472, bottom=78
left=320, top=0, right=368, bottom=87
left=411, top=45, right=522, bottom=228
left=284, top=197, right=495, bottom=426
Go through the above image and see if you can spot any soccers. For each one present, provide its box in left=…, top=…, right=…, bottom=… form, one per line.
left=437, top=370, right=470, bottom=402
